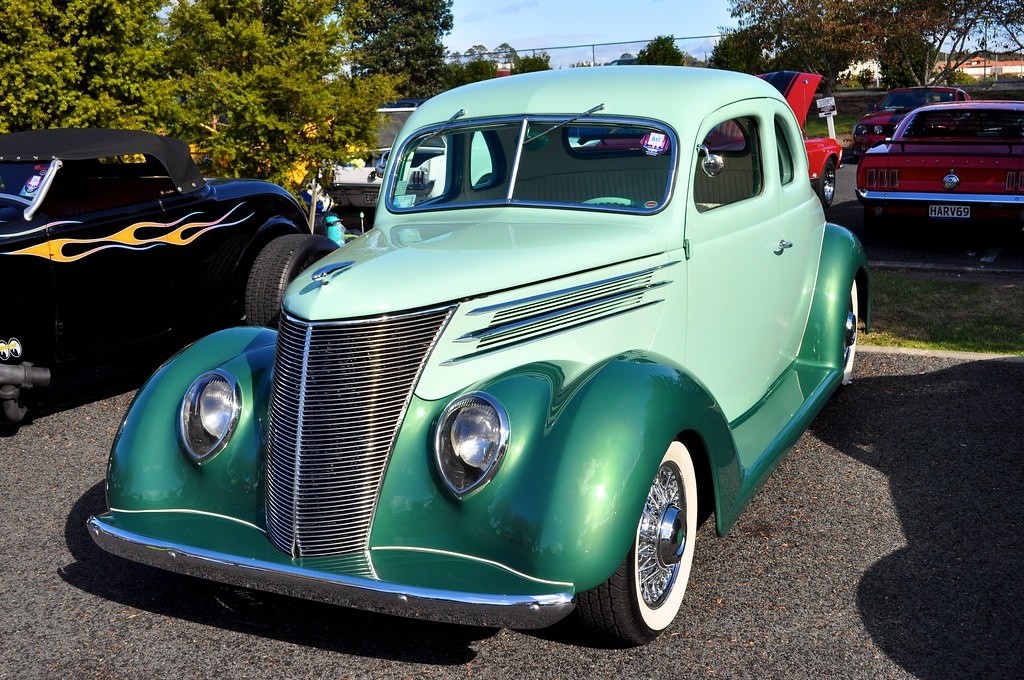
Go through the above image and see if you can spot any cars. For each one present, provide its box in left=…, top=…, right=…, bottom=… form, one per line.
left=0, top=128, right=341, bottom=423
left=318, top=99, right=493, bottom=218
left=854, top=99, right=1024, bottom=242
left=853, top=86, right=982, bottom=156
left=593, top=71, right=843, bottom=209
left=85, top=66, right=870, bottom=646
